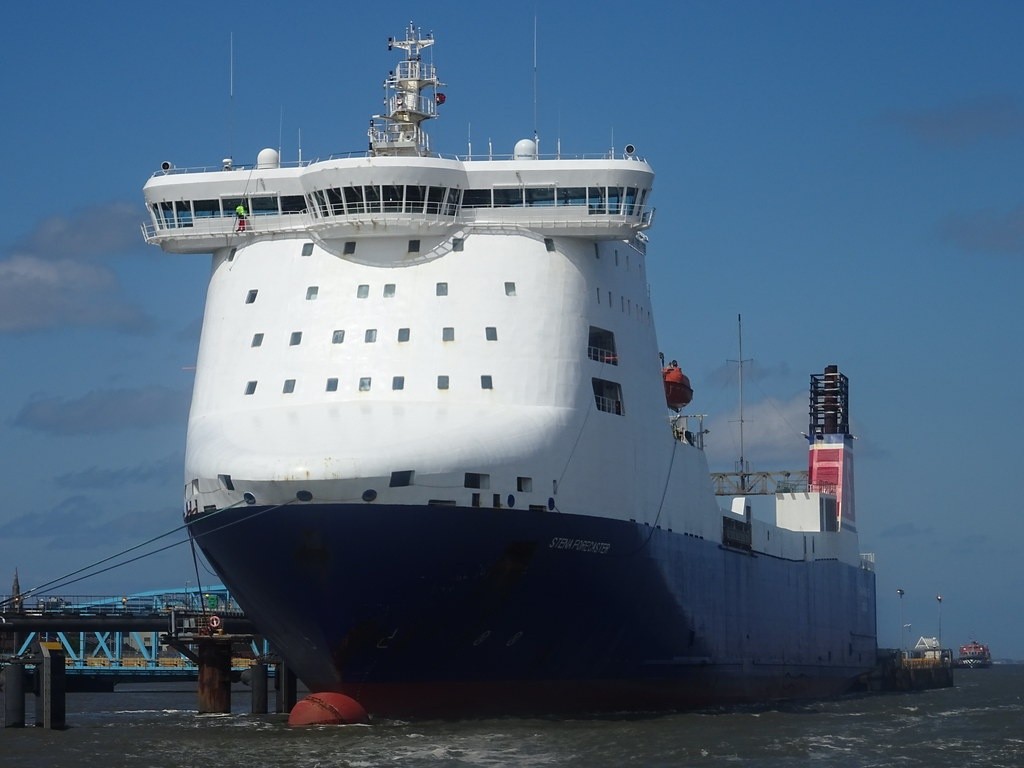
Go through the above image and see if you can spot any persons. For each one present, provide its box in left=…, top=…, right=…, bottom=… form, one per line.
left=236, top=202, right=248, bottom=232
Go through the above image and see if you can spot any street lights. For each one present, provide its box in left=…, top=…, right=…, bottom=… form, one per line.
left=936, top=592, right=944, bottom=649
left=896, top=588, right=904, bottom=650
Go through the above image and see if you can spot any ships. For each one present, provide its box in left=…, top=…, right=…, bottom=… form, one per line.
left=136, top=5, right=883, bottom=713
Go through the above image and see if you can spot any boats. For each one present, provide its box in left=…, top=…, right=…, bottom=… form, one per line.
left=902, top=649, right=954, bottom=689
left=954, top=640, right=992, bottom=669
